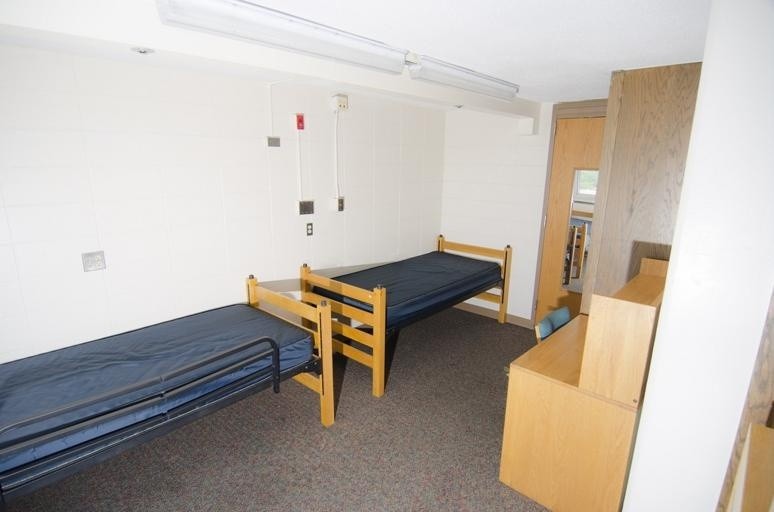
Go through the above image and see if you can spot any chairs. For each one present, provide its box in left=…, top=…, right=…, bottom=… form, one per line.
left=534, top=307, right=571, bottom=345
left=563, top=224, right=587, bottom=284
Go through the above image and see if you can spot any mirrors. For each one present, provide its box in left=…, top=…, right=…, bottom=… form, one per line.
left=562, top=169, right=598, bottom=293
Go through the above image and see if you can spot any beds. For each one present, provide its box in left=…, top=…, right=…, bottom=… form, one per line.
left=0, top=274, right=336, bottom=503
left=300, top=234, right=512, bottom=397
left=568, top=210, right=593, bottom=247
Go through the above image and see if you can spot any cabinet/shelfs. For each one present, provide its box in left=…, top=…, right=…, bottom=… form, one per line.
left=498, top=257, right=670, bottom=511
